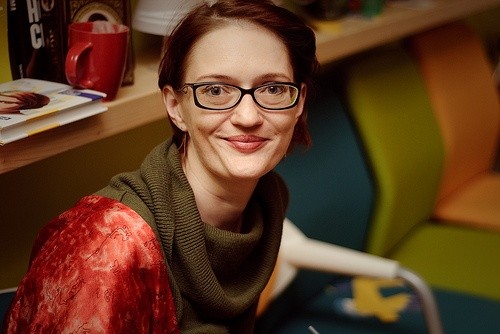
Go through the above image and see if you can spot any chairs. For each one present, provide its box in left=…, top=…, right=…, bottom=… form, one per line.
left=271, top=16, right=499, bottom=333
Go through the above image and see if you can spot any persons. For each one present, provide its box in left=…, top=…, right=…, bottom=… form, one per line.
left=1, top=0, right=319, bottom=334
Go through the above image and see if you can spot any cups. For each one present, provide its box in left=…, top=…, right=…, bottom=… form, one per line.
left=65, top=20, right=131, bottom=102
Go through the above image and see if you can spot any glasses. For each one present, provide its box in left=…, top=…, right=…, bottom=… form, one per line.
left=176, top=81, right=301, bottom=110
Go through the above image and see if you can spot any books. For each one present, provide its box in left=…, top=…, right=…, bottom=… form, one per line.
left=0, top=78, right=108, bottom=147
